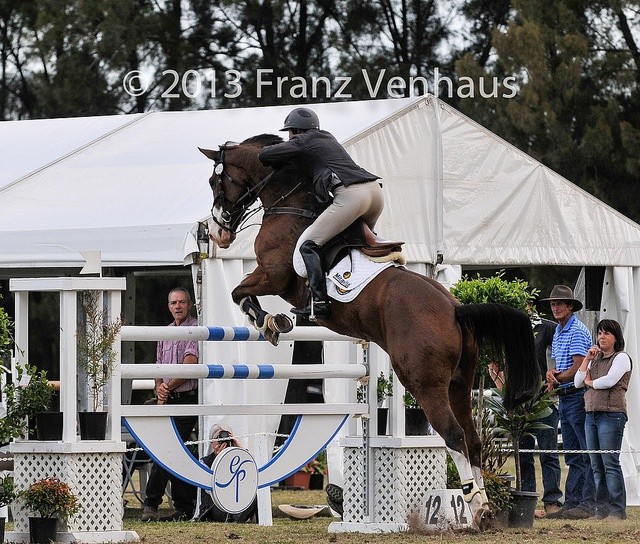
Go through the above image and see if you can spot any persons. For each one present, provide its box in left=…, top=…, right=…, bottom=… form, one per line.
left=199, top=424, right=256, bottom=523
left=141, top=287, right=198, bottom=521
left=574, top=319, right=633, bottom=521
left=538, top=285, right=597, bottom=519
left=488, top=303, right=563, bottom=516
left=258, top=108, right=384, bottom=320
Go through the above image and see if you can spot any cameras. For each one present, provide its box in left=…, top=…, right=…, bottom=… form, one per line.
left=218, top=431, right=232, bottom=442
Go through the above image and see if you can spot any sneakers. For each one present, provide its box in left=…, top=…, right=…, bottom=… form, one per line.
left=603, top=515, right=625, bottom=522
left=545, top=504, right=559, bottom=516
left=326, top=483, right=343, bottom=505
left=546, top=504, right=577, bottom=518
left=562, top=505, right=595, bottom=520
left=586, top=514, right=608, bottom=520
left=326, top=497, right=343, bottom=521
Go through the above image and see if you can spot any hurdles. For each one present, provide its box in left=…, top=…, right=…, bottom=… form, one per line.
left=5, top=275, right=448, bottom=543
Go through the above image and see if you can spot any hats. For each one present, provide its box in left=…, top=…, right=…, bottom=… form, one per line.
left=539, top=285, right=583, bottom=312
left=277, top=504, right=341, bottom=519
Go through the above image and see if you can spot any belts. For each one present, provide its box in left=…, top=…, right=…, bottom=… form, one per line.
left=557, top=388, right=584, bottom=395
left=170, top=391, right=195, bottom=397
left=332, top=182, right=343, bottom=191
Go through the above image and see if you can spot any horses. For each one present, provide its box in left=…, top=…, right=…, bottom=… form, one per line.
left=196, top=132, right=543, bottom=534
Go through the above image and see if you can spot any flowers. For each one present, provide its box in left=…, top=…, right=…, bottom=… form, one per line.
left=300, top=452, right=327, bottom=475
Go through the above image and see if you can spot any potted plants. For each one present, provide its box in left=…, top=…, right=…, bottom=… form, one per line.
left=386, top=266, right=549, bottom=530
left=388, top=370, right=431, bottom=436
left=77, top=290, right=129, bottom=441
left=0, top=364, right=63, bottom=448
left=1, top=473, right=20, bottom=543
left=378, top=374, right=390, bottom=436
left=16, top=476, right=81, bottom=543
left=484, top=376, right=565, bottom=530
left=446, top=402, right=514, bottom=529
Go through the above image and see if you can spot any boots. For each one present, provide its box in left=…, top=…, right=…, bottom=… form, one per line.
left=290, top=240, right=331, bottom=320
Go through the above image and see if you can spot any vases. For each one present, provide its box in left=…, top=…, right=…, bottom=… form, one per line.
left=311, top=475, right=323, bottom=490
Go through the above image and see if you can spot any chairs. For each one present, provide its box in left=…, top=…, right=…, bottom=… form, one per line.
left=123, top=395, right=172, bottom=508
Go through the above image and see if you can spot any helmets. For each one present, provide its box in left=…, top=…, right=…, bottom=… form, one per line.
left=279, top=108, right=319, bottom=131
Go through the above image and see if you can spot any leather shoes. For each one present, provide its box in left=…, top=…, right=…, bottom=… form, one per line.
left=160, top=510, right=194, bottom=521
left=142, top=506, right=159, bottom=522
